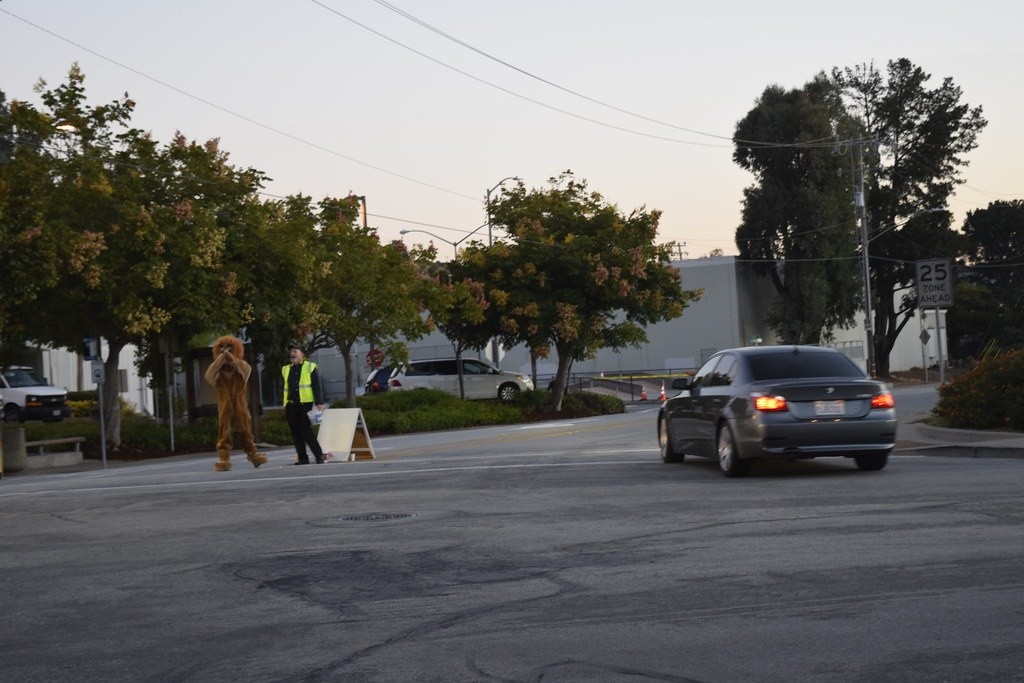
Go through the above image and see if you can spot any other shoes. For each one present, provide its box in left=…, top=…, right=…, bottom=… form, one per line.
left=294, top=458, right=309, bottom=465
left=316, top=457, right=325, bottom=464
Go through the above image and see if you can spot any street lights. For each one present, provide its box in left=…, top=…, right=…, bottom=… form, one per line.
left=486, top=175, right=520, bottom=371
left=398, top=220, right=496, bottom=263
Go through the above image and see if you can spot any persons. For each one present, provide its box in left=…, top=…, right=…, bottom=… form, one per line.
left=280, top=343, right=328, bottom=466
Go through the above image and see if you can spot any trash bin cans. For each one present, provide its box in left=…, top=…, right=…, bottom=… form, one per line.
left=0, top=424, right=27, bottom=472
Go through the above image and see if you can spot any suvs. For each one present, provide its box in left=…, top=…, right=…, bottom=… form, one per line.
left=364, top=362, right=405, bottom=396
left=386, top=356, right=534, bottom=405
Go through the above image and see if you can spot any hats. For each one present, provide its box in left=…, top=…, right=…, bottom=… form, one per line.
left=287, top=343, right=305, bottom=353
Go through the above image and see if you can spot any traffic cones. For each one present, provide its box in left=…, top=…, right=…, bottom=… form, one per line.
left=659, top=379, right=667, bottom=400
left=640, top=385, right=648, bottom=401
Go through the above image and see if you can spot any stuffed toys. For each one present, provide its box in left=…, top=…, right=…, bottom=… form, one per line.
left=205, top=333, right=268, bottom=471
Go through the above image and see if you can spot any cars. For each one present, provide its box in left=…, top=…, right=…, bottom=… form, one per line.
left=656, top=344, right=899, bottom=475
left=0, top=364, right=70, bottom=424
